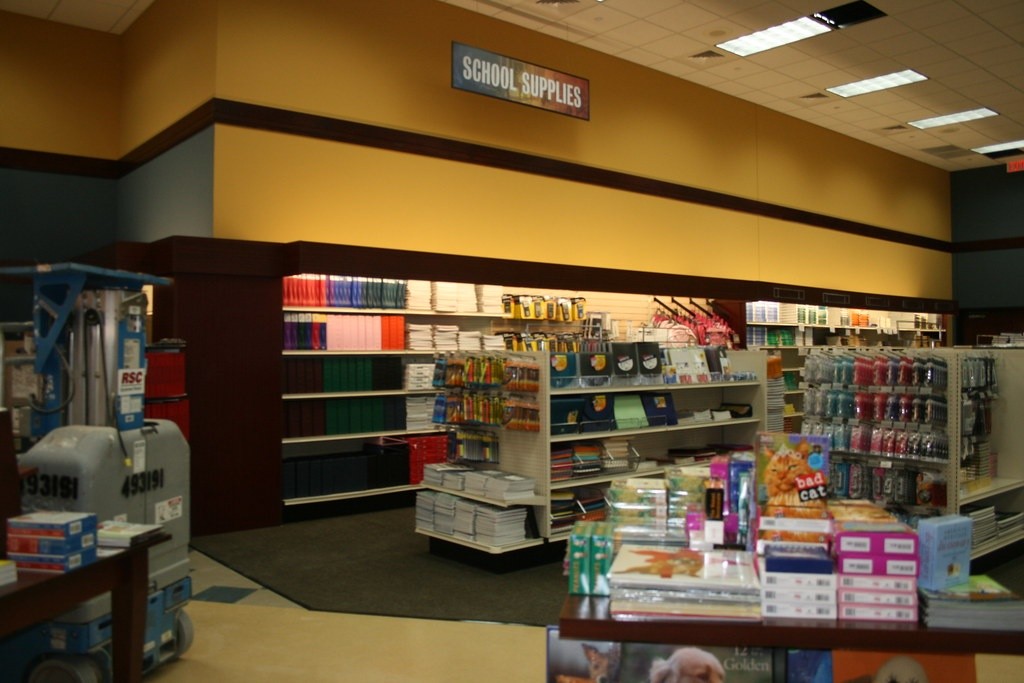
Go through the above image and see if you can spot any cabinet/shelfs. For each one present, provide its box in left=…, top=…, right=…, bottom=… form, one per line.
left=414, top=349, right=547, bottom=575
left=281, top=305, right=512, bottom=524
left=902, top=347, right=1024, bottom=562
left=747, top=321, right=946, bottom=419
left=546, top=349, right=767, bottom=564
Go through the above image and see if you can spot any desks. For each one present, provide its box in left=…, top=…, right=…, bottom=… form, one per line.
left=558, top=544, right=1024, bottom=656
left=0, top=533, right=172, bottom=683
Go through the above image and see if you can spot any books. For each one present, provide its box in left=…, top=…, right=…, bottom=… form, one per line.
left=607, top=543, right=762, bottom=624
left=550, top=347, right=753, bottom=535
left=960, top=504, right=1021, bottom=546
left=415, top=462, right=537, bottom=547
left=918, top=575, right=1024, bottom=630
left=281, top=273, right=506, bottom=438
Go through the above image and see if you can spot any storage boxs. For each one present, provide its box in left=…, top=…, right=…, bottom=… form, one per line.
left=663, top=466, right=710, bottom=493
left=7, top=529, right=98, bottom=556
left=667, top=509, right=686, bottom=528
left=7, top=510, right=97, bottom=539
left=614, top=523, right=667, bottom=537
left=7, top=545, right=97, bottom=574
left=568, top=520, right=594, bottom=595
left=728, top=451, right=756, bottom=534
left=590, top=521, right=617, bottom=597
left=750, top=430, right=973, bottom=622
left=667, top=490, right=705, bottom=509
left=608, top=478, right=667, bottom=506
left=711, top=454, right=730, bottom=516
left=609, top=505, right=667, bottom=524
